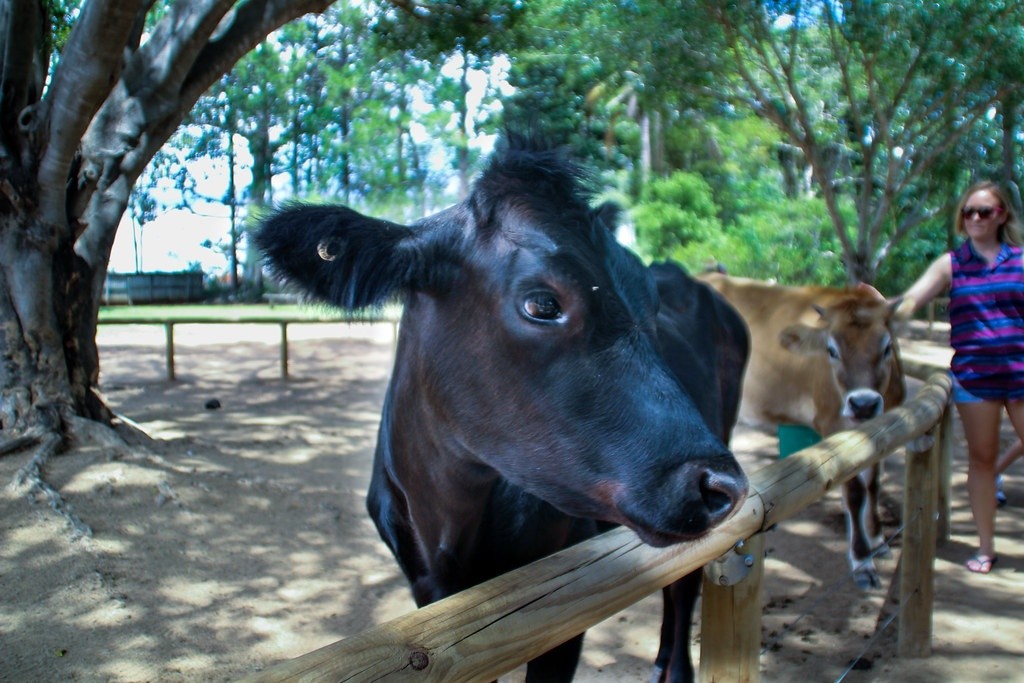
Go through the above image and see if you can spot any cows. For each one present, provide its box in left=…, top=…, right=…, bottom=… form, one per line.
left=690, top=270, right=907, bottom=592
left=253, top=140, right=751, bottom=683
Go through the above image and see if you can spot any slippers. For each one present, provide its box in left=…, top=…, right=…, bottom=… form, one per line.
left=966, top=551, right=998, bottom=573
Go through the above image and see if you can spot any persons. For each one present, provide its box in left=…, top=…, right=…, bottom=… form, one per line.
left=890, top=181, right=1024, bottom=572
left=705, top=255, right=728, bottom=274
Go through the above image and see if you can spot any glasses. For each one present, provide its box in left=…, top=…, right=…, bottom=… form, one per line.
left=961, top=206, right=1004, bottom=220
